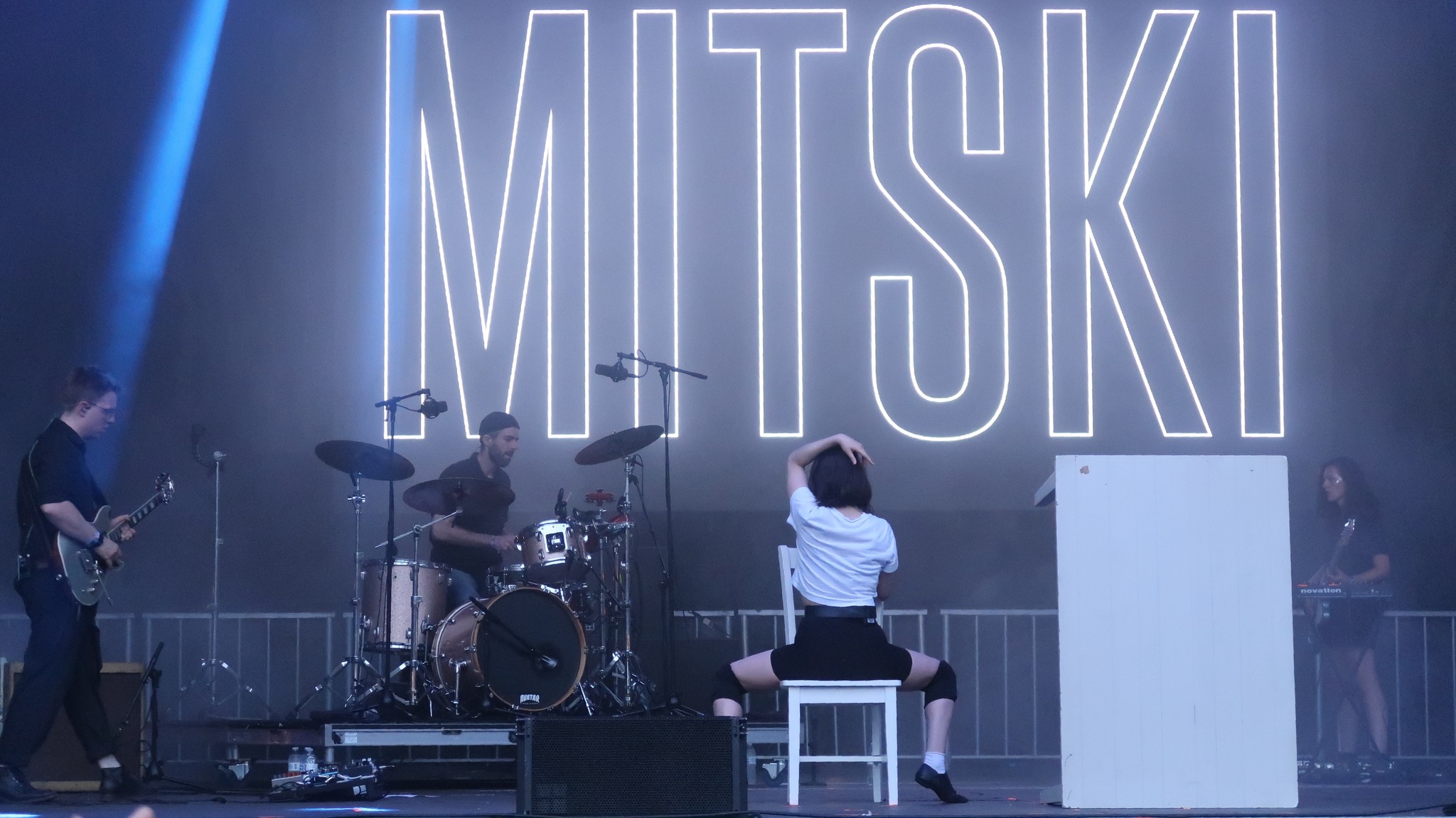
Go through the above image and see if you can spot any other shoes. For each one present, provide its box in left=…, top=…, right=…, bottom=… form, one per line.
left=915, top=762, right=968, bottom=804
left=1, top=771, right=57, bottom=804
left=98, top=772, right=162, bottom=801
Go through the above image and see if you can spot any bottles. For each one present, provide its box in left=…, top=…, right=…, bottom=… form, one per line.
left=288, top=747, right=302, bottom=777
left=302, top=747, right=318, bottom=774
left=746, top=743, right=757, bottom=784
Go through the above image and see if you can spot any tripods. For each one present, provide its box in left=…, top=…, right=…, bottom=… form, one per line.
left=116, top=642, right=213, bottom=793
left=565, top=352, right=708, bottom=718
left=285, top=388, right=463, bottom=720
left=167, top=453, right=281, bottom=721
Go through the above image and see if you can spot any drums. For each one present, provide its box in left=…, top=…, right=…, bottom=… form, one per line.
left=485, top=565, right=535, bottom=597
left=430, top=587, right=587, bottom=712
left=518, top=519, right=590, bottom=584
left=361, top=559, right=452, bottom=656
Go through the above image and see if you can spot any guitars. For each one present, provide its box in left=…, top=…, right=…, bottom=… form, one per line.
left=53, top=473, right=175, bottom=606
left=1313, top=518, right=1356, bottom=627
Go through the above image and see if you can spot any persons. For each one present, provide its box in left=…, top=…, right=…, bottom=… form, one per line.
left=429, top=410, right=524, bottom=605
left=1307, top=457, right=1398, bottom=759
left=712, top=433, right=968, bottom=803
left=0, top=365, right=160, bottom=804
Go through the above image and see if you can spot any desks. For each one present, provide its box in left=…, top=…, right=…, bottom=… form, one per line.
left=1034, top=448, right=1303, bottom=811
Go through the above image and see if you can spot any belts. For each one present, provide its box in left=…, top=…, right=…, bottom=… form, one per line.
left=804, top=606, right=877, bottom=619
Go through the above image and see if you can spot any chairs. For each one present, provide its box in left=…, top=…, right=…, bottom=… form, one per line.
left=774, top=538, right=907, bottom=808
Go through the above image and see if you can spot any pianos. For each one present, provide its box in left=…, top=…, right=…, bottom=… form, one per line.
left=1293, top=580, right=1395, bottom=784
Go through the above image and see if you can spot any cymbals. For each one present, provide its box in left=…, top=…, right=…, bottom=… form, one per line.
left=403, top=478, right=516, bottom=517
left=586, top=522, right=617, bottom=526
left=575, top=424, right=664, bottom=465
left=315, top=440, right=415, bottom=481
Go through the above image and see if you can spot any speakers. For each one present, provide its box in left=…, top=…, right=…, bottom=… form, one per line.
left=513, top=716, right=747, bottom=818
left=3, top=660, right=145, bottom=791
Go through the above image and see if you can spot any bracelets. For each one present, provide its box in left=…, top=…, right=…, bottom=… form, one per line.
left=88, top=531, right=107, bottom=549
left=491, top=535, right=496, bottom=552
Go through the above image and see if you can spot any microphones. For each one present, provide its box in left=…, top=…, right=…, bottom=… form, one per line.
left=418, top=401, right=448, bottom=414
left=539, top=655, right=559, bottom=671
left=617, top=455, right=637, bottom=513
left=555, top=489, right=563, bottom=516
left=594, top=364, right=639, bottom=379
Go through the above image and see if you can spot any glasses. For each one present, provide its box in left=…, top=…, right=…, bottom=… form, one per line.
left=88, top=401, right=116, bottom=416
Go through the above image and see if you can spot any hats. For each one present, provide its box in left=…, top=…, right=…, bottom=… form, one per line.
left=478, top=411, right=519, bottom=436
